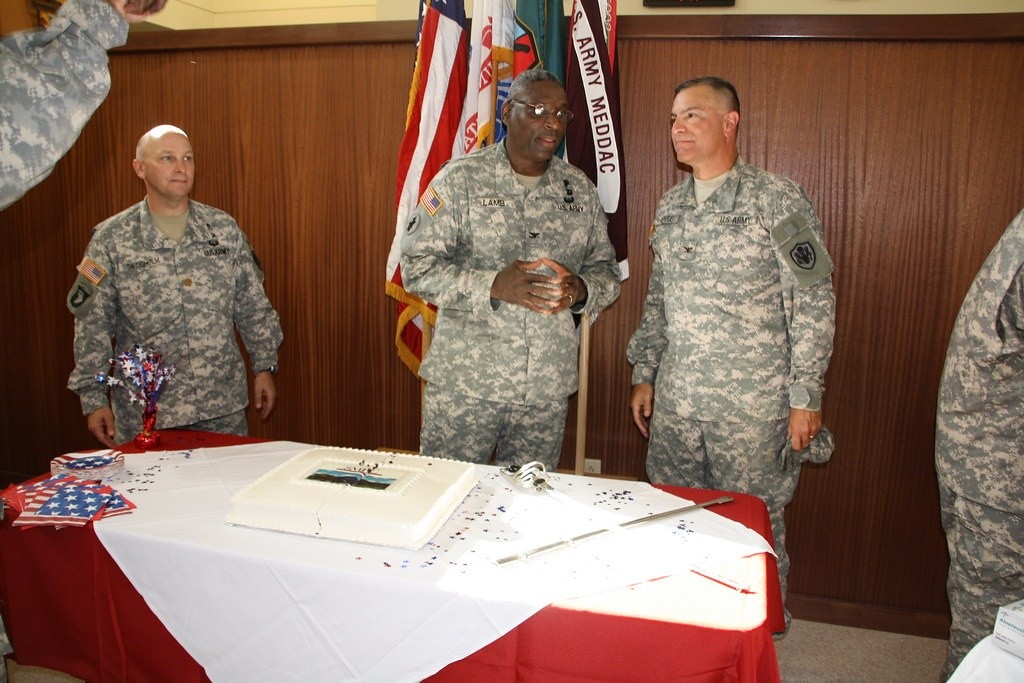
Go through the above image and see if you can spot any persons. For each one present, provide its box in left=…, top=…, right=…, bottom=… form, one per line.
left=625, top=77, right=836, bottom=638
left=400, top=68, right=622, bottom=473
left=65, top=124, right=284, bottom=449
left=937, top=207, right=1024, bottom=683
left=0, top=0, right=167, bottom=212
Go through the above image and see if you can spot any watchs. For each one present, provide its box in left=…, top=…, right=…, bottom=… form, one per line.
left=261, top=366, right=276, bottom=374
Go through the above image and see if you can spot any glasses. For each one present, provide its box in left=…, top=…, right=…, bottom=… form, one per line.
left=506, top=98, right=574, bottom=124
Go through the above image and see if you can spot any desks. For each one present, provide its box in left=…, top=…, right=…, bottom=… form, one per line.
left=0, top=428, right=786, bottom=683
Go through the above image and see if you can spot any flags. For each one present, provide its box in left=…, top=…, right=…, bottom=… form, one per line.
left=385, top=0, right=630, bottom=383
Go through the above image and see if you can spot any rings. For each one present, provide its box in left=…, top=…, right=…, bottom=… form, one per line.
left=569, top=294, right=572, bottom=304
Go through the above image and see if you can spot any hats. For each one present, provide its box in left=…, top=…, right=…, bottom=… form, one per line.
left=780, top=424, right=834, bottom=473
